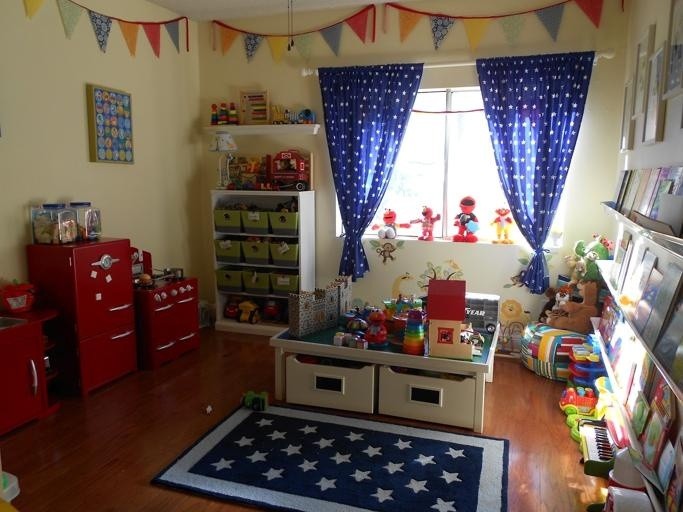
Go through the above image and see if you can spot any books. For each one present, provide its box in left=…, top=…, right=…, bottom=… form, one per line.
left=597, top=167, right=683, bottom=512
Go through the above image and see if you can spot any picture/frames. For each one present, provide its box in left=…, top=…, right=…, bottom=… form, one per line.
left=86, top=83, right=134, bottom=164
left=620, top=0, right=683, bottom=154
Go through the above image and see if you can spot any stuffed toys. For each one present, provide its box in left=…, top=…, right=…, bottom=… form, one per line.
left=538, top=235, right=615, bottom=334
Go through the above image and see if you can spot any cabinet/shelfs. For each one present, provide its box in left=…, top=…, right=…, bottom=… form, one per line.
left=210, top=189, right=316, bottom=337
left=590, top=200, right=683, bottom=512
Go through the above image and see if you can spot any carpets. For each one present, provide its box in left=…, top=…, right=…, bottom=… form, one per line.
left=150, top=403, right=510, bottom=512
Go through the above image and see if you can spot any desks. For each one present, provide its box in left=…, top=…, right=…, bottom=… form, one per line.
left=269, top=316, right=501, bottom=433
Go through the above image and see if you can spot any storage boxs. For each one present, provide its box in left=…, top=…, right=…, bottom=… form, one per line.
left=215, top=210, right=299, bottom=294
left=285, top=354, right=377, bottom=414
left=378, top=365, right=476, bottom=429
